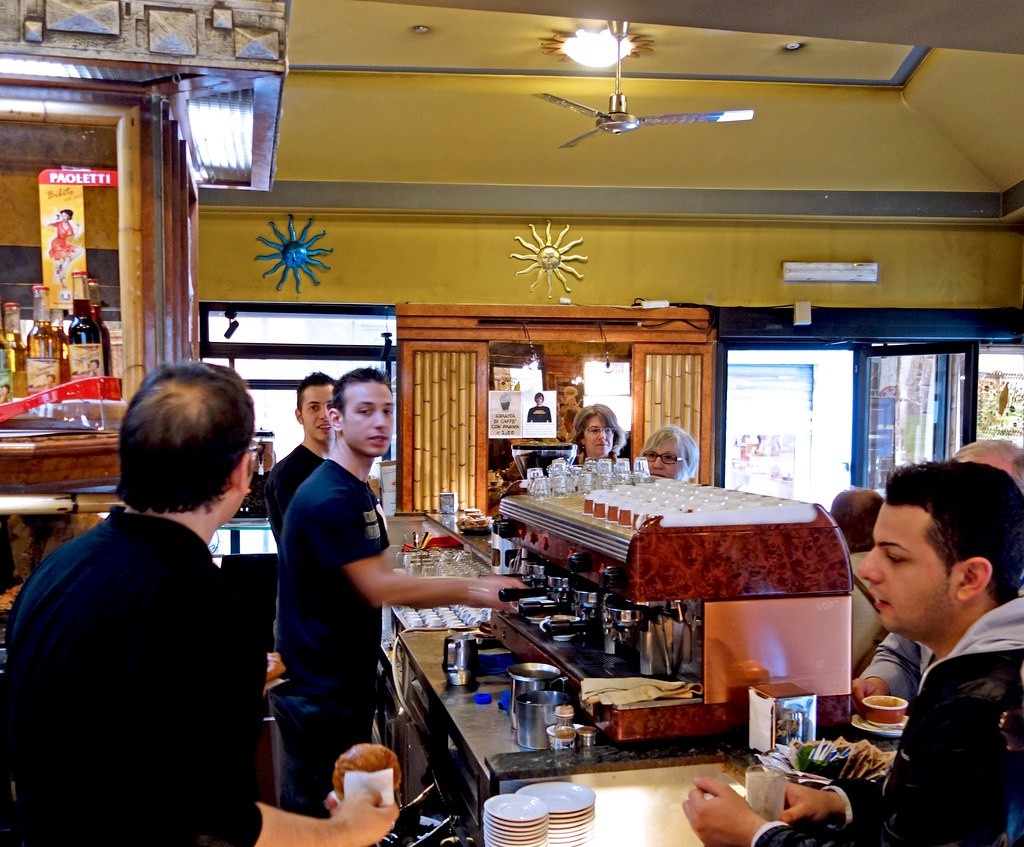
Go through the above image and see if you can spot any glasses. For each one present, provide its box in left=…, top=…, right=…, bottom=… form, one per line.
left=233, top=441, right=263, bottom=461
left=583, top=427, right=616, bottom=435
left=643, top=450, right=690, bottom=464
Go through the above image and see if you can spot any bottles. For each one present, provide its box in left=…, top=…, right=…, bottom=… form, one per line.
left=553, top=705, right=576, bottom=750
left=395, top=552, right=498, bottom=633
left=1, top=271, right=112, bottom=405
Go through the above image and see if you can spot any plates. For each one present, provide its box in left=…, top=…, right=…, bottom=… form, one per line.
left=849, top=712, right=911, bottom=737
left=483, top=781, right=597, bottom=847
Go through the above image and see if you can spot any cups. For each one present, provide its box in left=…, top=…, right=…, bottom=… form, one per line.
left=526, top=454, right=807, bottom=531
left=506, top=663, right=599, bottom=751
left=745, top=763, right=787, bottom=824
left=862, top=695, right=909, bottom=726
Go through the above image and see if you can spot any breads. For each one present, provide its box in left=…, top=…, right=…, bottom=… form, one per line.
left=333, top=742, right=400, bottom=800
left=266, top=652, right=285, bottom=682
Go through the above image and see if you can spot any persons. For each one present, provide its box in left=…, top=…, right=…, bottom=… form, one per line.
left=566, top=405, right=626, bottom=466
left=528, top=393, right=552, bottom=423
left=642, top=426, right=698, bottom=480
left=1, top=365, right=399, bottom=847
left=831, top=490, right=890, bottom=676
left=684, top=461, right=1024, bottom=847
left=851, top=440, right=1024, bottom=720
left=270, top=368, right=528, bottom=819
left=264, top=374, right=339, bottom=544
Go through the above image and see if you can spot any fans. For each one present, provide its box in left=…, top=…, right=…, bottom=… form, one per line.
left=532, top=18, right=756, bottom=149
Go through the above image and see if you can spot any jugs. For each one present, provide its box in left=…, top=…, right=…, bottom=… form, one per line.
left=441, top=632, right=478, bottom=686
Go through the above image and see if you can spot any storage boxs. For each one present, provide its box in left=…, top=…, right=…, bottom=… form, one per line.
left=748, top=683, right=817, bottom=753
left=439, top=492, right=459, bottom=515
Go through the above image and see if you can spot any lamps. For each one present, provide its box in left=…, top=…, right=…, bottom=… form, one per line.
left=597, top=323, right=613, bottom=373
left=522, top=321, right=543, bottom=370
left=380, top=332, right=392, bottom=360
left=782, top=260, right=879, bottom=283
left=224, top=310, right=239, bottom=340
left=640, top=300, right=671, bottom=310
